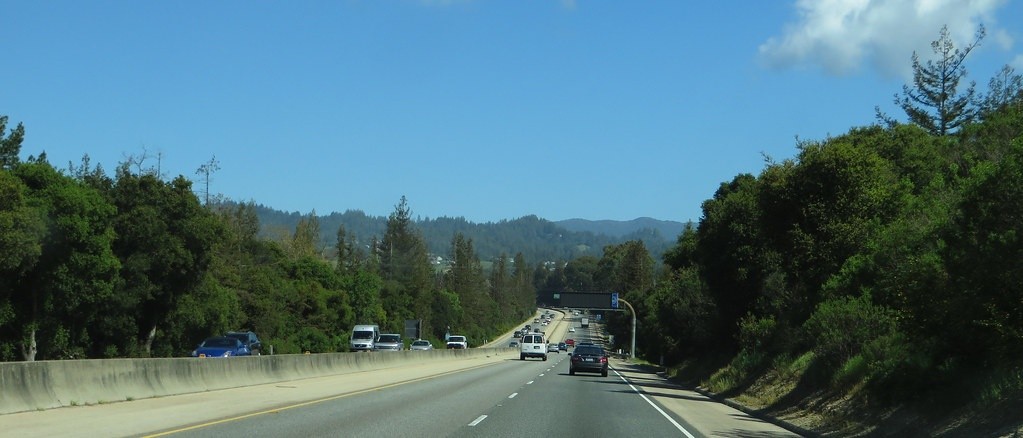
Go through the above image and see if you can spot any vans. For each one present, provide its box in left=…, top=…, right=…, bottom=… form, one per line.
left=520, top=333, right=549, bottom=361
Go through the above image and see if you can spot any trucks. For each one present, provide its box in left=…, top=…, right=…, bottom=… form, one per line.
left=580, top=318, right=589, bottom=328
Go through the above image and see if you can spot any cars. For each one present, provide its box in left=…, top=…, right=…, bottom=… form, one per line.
left=534, top=328, right=540, bottom=333
left=525, top=325, right=531, bottom=330
left=565, top=339, right=575, bottom=348
left=559, top=342, right=567, bottom=352
left=541, top=315, right=545, bottom=319
left=547, top=343, right=559, bottom=353
left=542, top=321, right=547, bottom=326
left=509, top=342, right=519, bottom=347
left=191, top=324, right=467, bottom=358
left=546, top=317, right=551, bottom=322
left=550, top=314, right=555, bottom=318
left=569, top=309, right=578, bottom=316
left=568, top=344, right=610, bottom=377
left=546, top=311, right=549, bottom=314
left=534, top=318, right=540, bottom=323
left=521, top=328, right=528, bottom=333
left=513, top=331, right=522, bottom=338
left=569, top=328, right=575, bottom=333
